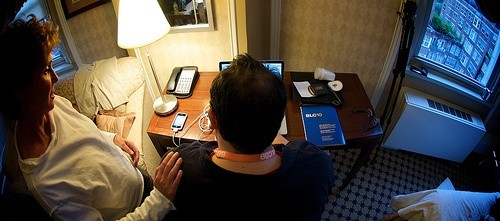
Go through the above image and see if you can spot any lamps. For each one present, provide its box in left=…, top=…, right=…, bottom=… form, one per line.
left=116, top=0, right=177, bottom=116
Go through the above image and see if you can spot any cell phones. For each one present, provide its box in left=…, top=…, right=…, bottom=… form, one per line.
left=171, top=112, right=187, bottom=130
left=308, top=84, right=328, bottom=95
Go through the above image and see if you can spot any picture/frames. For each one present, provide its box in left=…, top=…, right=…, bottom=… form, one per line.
left=158, top=0, right=215, bottom=33
left=62, top=0, right=111, bottom=21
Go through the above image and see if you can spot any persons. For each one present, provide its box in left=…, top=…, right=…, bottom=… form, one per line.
left=158, top=55, right=335, bottom=221
left=0, top=14, right=182, bottom=221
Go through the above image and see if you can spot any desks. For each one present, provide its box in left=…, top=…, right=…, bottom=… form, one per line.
left=147, top=71, right=383, bottom=188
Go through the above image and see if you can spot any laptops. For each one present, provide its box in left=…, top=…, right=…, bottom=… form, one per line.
left=219, top=60, right=288, bottom=135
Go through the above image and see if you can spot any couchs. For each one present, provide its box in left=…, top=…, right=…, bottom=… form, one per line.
left=53, top=56, right=162, bottom=181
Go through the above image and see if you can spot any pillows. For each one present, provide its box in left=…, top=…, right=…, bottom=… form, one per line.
left=95, top=114, right=124, bottom=138
left=99, top=109, right=136, bottom=138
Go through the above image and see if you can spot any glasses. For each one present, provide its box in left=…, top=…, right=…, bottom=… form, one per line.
left=351, top=108, right=378, bottom=135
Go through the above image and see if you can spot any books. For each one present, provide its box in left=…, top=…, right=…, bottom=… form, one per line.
left=300, top=106, right=346, bottom=146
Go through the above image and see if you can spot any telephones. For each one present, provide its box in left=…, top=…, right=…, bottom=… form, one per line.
left=165, top=65, right=200, bottom=97
